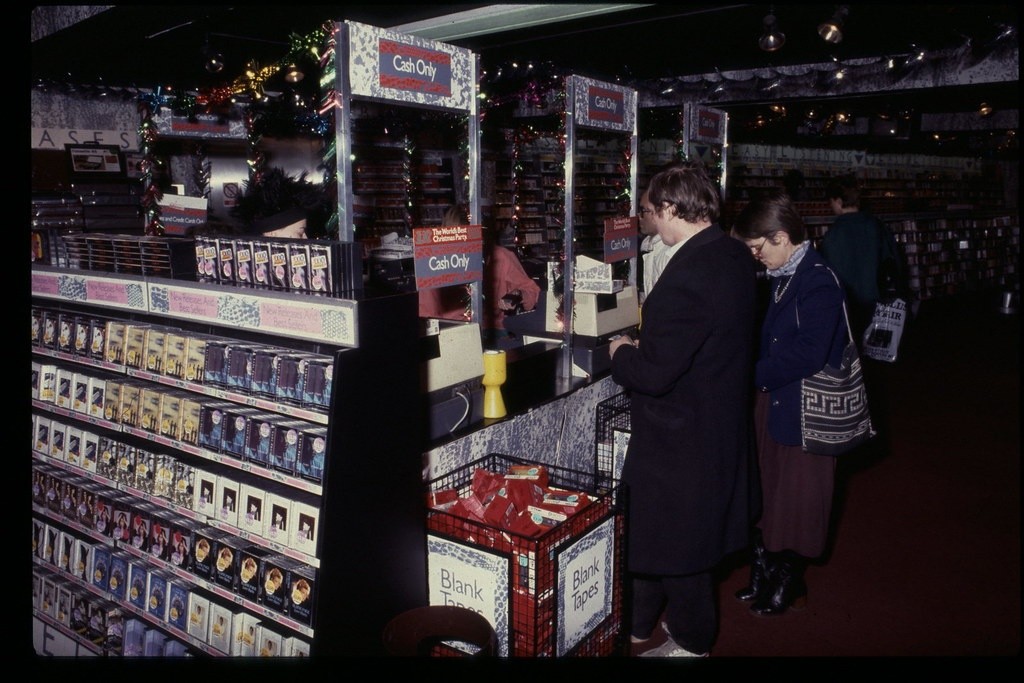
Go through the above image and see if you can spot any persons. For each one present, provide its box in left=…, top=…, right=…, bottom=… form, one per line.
left=261, top=207, right=308, bottom=238
left=96, top=506, right=309, bottom=601
left=418, top=205, right=540, bottom=331
left=607, top=161, right=911, bottom=656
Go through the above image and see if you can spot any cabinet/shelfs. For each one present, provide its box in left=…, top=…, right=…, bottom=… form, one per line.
left=32, top=262, right=360, bottom=656
left=350, top=131, right=1024, bottom=306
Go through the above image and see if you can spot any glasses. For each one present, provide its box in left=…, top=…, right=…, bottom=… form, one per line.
left=640, top=209, right=655, bottom=218
left=748, top=233, right=772, bottom=252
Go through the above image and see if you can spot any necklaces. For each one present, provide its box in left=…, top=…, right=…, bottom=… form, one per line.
left=774, top=273, right=794, bottom=304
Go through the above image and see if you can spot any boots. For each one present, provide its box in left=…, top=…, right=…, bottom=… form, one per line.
left=734, top=540, right=773, bottom=602
left=750, top=553, right=807, bottom=617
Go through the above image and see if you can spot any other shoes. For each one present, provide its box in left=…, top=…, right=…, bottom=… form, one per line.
left=637, top=621, right=709, bottom=657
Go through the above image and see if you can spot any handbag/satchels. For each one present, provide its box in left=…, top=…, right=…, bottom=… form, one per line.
left=862, top=298, right=907, bottom=363
left=801, top=341, right=877, bottom=456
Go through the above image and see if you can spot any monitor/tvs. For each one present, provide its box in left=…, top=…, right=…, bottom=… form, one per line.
left=63, top=143, right=127, bottom=176
